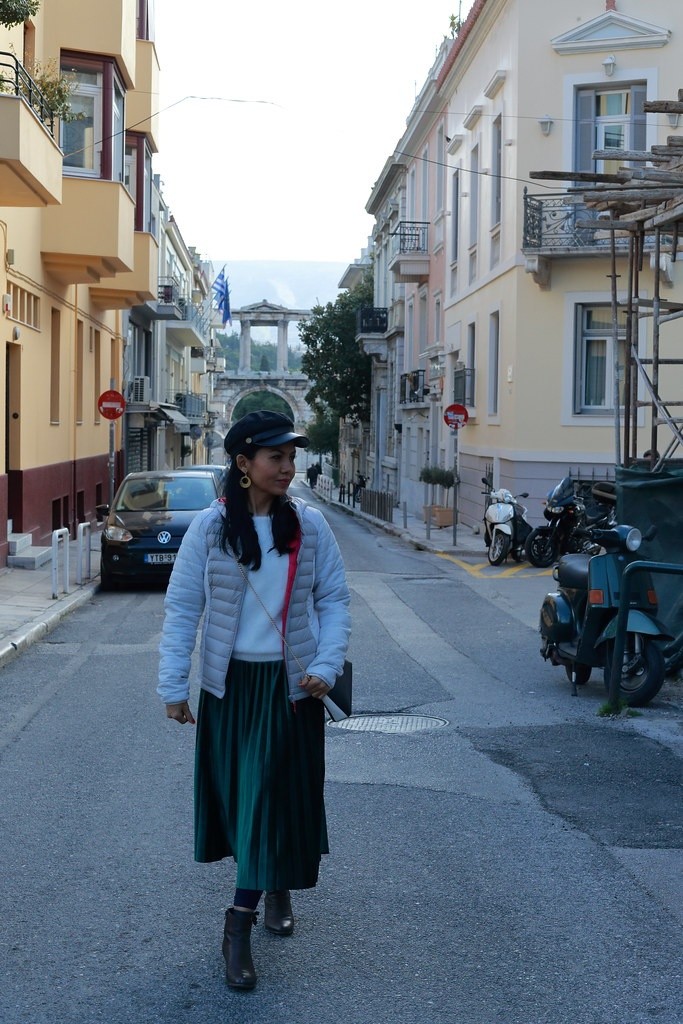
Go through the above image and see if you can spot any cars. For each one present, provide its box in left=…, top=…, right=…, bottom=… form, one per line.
left=94, top=465, right=230, bottom=587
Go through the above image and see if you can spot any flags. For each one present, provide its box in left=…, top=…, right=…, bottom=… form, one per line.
left=211, top=268, right=233, bottom=329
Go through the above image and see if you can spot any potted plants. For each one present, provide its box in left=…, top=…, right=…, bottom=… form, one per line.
left=418, top=465, right=445, bottom=524
left=434, top=468, right=462, bottom=527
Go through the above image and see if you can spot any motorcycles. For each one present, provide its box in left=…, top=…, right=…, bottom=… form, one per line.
left=524, top=475, right=613, bottom=568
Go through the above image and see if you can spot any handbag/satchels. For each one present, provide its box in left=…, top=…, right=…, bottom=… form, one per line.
left=321, top=659, right=352, bottom=722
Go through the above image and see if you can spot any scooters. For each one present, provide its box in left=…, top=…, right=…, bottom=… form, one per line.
left=354, top=470, right=370, bottom=502
left=540, top=525, right=675, bottom=707
left=480, top=477, right=529, bottom=565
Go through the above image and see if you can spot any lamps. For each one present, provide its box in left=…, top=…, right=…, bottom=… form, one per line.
left=414, top=383, right=436, bottom=395
left=408, top=413, right=428, bottom=422
left=665, top=113, right=682, bottom=129
left=602, top=55, right=617, bottom=77
left=538, top=114, right=554, bottom=137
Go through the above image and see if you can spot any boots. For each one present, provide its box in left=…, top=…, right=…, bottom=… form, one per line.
left=222, top=908, right=260, bottom=987
left=264, top=890, right=294, bottom=934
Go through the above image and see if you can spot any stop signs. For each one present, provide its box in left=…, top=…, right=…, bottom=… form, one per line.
left=445, top=404, right=469, bottom=429
left=96, top=388, right=124, bottom=420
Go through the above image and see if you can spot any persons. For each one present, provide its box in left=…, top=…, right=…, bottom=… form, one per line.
left=643, top=450, right=661, bottom=458
left=157, top=410, right=349, bottom=990
left=307, top=461, right=366, bottom=499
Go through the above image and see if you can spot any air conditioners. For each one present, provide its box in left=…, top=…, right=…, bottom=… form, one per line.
left=133, top=376, right=150, bottom=402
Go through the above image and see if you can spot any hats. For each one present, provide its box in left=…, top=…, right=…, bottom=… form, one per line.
left=224, top=411, right=310, bottom=457
